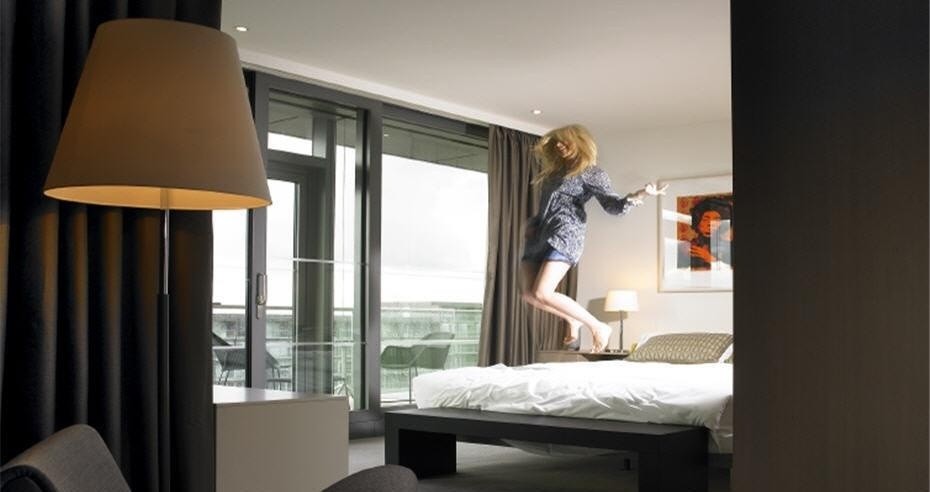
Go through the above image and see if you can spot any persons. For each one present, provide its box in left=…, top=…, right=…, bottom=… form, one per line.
left=515, top=122, right=670, bottom=353
left=677, top=195, right=735, bottom=270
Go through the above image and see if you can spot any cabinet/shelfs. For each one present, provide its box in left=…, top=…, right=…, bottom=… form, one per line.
left=212, top=385, right=350, bottom=490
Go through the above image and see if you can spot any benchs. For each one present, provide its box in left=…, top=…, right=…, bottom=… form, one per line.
left=383, top=407, right=710, bottom=492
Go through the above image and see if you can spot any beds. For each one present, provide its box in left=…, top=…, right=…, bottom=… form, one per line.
left=416, top=328, right=737, bottom=454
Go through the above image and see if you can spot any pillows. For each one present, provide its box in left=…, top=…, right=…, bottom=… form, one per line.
left=624, top=330, right=736, bottom=363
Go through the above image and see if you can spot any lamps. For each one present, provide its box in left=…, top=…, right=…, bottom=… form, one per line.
left=38, top=14, right=275, bottom=490
left=603, top=286, right=640, bottom=351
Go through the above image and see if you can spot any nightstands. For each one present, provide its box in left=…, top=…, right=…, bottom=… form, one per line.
left=534, top=350, right=628, bottom=364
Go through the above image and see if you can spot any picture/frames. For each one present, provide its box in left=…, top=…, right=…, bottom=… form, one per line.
left=655, top=169, right=743, bottom=297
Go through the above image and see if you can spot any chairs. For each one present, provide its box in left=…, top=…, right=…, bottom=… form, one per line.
left=206, top=322, right=280, bottom=389
left=379, top=330, right=456, bottom=403
left=2, top=419, right=424, bottom=492
